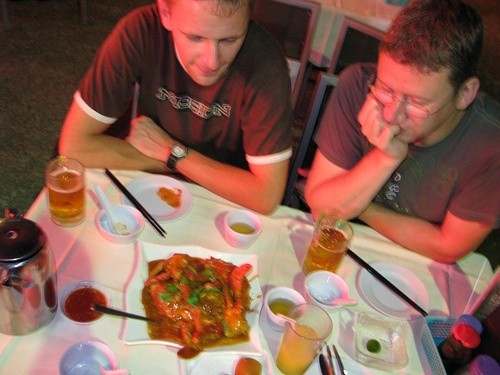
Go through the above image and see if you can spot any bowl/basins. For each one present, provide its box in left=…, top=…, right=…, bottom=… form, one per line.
left=96, top=205, right=144, bottom=244
left=355, top=310, right=409, bottom=370
left=303, top=270, right=350, bottom=311
left=58, top=280, right=111, bottom=325
left=59, top=339, right=119, bottom=375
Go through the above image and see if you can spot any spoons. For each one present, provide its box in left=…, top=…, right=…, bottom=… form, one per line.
left=91, top=182, right=129, bottom=236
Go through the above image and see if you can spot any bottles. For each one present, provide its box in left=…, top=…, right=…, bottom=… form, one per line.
left=439, top=314, right=500, bottom=375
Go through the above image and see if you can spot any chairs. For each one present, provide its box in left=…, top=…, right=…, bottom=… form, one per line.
left=251, top=0, right=387, bottom=211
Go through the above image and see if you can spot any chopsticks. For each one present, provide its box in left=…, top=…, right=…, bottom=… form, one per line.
left=105, top=168, right=168, bottom=238
left=90, top=303, right=153, bottom=322
left=346, top=249, right=428, bottom=318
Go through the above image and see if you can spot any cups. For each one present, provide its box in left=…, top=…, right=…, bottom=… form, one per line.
left=264, top=286, right=306, bottom=332
left=276, top=303, right=333, bottom=375
left=223, top=209, right=262, bottom=249
left=302, top=215, right=355, bottom=276
left=44, top=154, right=86, bottom=228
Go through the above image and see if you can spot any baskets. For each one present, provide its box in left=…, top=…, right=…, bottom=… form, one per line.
left=421, top=316, right=458, bottom=375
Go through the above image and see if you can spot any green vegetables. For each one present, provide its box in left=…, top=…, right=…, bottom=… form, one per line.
left=159, top=265, right=221, bottom=307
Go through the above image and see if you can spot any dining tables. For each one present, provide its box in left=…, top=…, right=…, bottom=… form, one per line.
left=0, top=168, right=494, bottom=375
left=309, top=0, right=403, bottom=69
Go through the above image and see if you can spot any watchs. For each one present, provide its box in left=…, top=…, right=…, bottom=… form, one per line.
left=167, top=140, right=190, bottom=175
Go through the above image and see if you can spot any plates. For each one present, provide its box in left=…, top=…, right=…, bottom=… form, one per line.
left=119, top=239, right=266, bottom=358
left=357, top=261, right=430, bottom=320
left=122, top=174, right=192, bottom=223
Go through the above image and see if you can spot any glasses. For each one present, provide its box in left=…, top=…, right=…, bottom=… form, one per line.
left=365, top=73, right=458, bottom=118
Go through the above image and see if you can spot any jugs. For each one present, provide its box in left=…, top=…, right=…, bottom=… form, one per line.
left=0, top=206, right=60, bottom=336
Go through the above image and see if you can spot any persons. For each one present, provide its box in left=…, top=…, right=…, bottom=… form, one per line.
left=57, top=0, right=293, bottom=215
left=293, top=0, right=500, bottom=266
